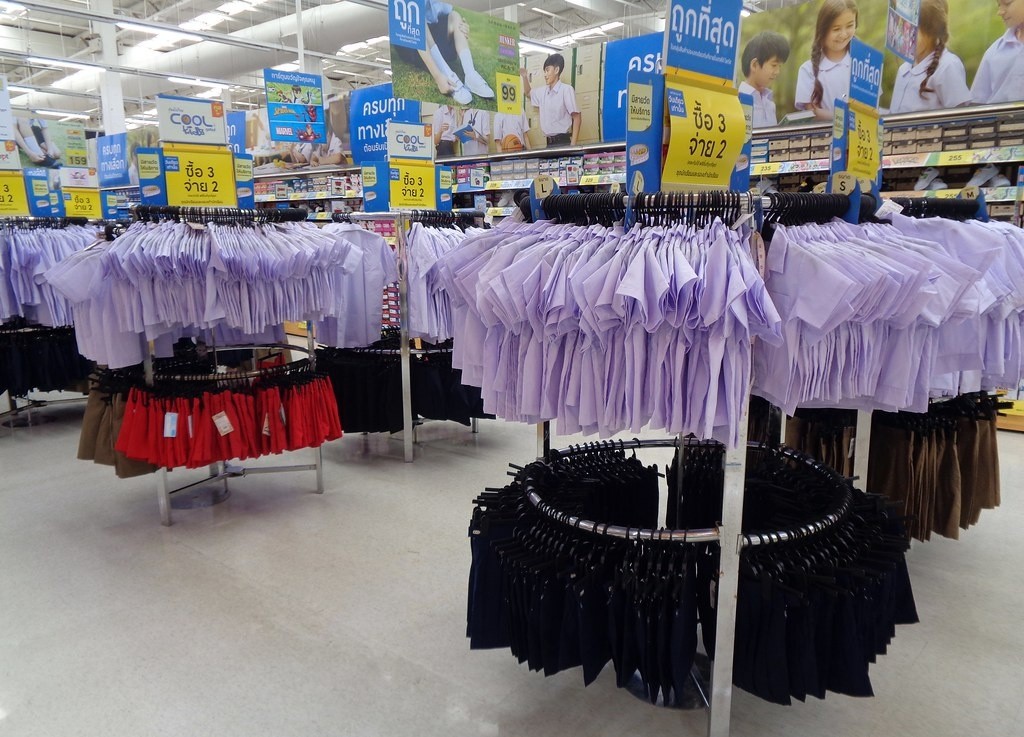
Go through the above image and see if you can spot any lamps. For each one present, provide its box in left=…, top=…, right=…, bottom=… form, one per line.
left=0, top=0, right=624, bottom=134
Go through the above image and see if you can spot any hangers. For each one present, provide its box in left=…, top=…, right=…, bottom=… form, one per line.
left=80, top=341, right=330, bottom=412
left=0, top=320, right=75, bottom=347
left=327, top=325, right=454, bottom=364
left=506, top=184, right=982, bottom=234
left=471, top=392, right=1017, bottom=621
left=0, top=205, right=489, bottom=234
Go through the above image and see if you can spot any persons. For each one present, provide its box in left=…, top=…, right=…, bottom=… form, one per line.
left=432, top=103, right=460, bottom=158
left=391, top=0, right=495, bottom=105
left=518, top=53, right=582, bottom=152
left=124, top=144, right=142, bottom=188
left=735, top=29, right=790, bottom=130
left=966, top=0, right=1024, bottom=108
left=247, top=85, right=348, bottom=167
left=794, top=1, right=885, bottom=122
left=12, top=117, right=60, bottom=167
left=889, top=0, right=974, bottom=118
left=493, top=105, right=532, bottom=156
left=144, top=130, right=155, bottom=148
left=458, top=105, right=492, bottom=157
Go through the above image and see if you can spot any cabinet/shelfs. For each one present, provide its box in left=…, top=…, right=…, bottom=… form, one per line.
left=579, top=146, right=1024, bottom=435
left=254, top=149, right=583, bottom=236
